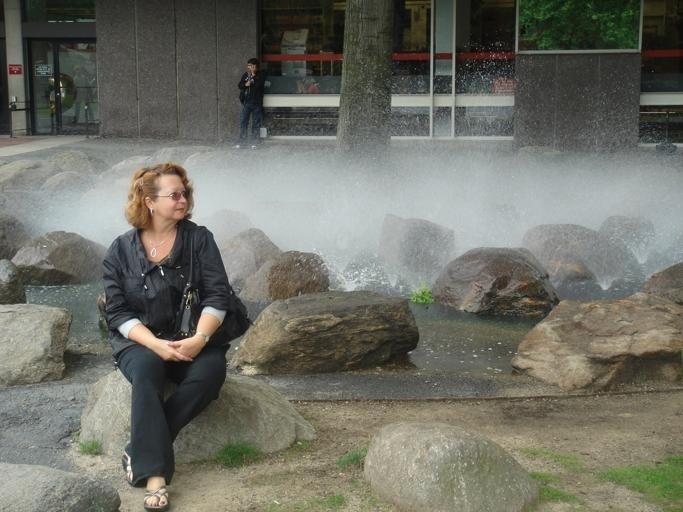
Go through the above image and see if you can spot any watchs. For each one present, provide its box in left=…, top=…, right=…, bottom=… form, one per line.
left=195, top=331, right=210, bottom=343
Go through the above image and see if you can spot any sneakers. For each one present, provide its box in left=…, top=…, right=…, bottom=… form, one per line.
left=234, top=143, right=244, bottom=149
left=248, top=145, right=258, bottom=149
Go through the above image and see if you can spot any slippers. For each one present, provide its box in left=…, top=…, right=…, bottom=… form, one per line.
left=144, top=486, right=170, bottom=512
left=122, top=455, right=146, bottom=487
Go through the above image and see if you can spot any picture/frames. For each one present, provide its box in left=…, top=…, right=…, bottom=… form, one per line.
left=515, top=0, right=643, bottom=54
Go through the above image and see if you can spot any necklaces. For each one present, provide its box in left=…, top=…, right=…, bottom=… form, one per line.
left=145, top=230, right=174, bottom=257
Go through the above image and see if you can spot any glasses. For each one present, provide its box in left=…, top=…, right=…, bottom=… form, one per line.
left=155, top=192, right=187, bottom=200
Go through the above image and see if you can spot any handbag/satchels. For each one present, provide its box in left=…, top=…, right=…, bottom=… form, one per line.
left=174, top=281, right=253, bottom=346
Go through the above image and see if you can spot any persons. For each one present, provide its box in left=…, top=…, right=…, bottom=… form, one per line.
left=71, top=69, right=96, bottom=123
left=100, top=160, right=236, bottom=511
left=231, top=58, right=267, bottom=149
